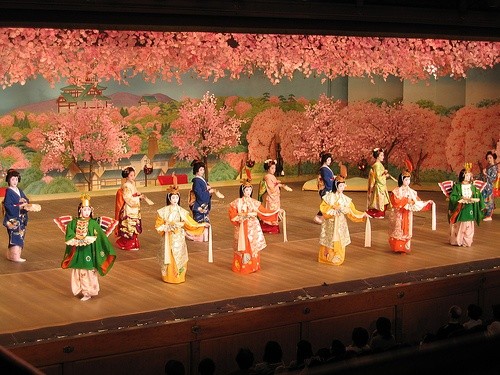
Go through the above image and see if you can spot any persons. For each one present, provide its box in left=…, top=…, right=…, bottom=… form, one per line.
left=438, top=162, right=487, bottom=246
left=154, top=187, right=210, bottom=283
left=114, top=167, right=145, bottom=250
left=188, top=159, right=215, bottom=241
left=318, top=175, right=372, bottom=266
left=388, top=169, right=436, bottom=255
left=365, top=148, right=389, bottom=219
left=312, top=151, right=335, bottom=225
left=228, top=179, right=287, bottom=276
left=163, top=316, right=393, bottom=375
left=479, top=148, right=500, bottom=222
left=54, top=193, right=119, bottom=301
left=428, top=300, right=500, bottom=338
left=0, top=169, right=31, bottom=262
left=256, top=159, right=293, bottom=234
